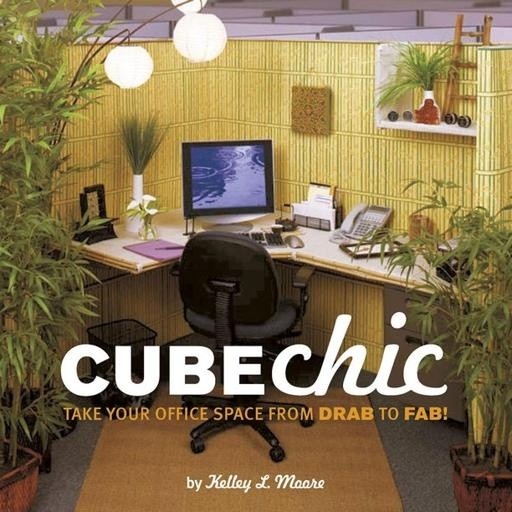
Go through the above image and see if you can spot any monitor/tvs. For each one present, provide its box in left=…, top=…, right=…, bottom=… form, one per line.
left=182, top=140, right=274, bottom=234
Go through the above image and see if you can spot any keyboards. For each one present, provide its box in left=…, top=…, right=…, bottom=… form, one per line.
left=238, top=232, right=292, bottom=255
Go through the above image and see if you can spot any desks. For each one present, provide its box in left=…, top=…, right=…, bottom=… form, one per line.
left=45, top=203, right=475, bottom=427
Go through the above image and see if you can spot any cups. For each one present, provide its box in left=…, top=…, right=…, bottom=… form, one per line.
left=408, top=214, right=428, bottom=255
left=280, top=202, right=294, bottom=227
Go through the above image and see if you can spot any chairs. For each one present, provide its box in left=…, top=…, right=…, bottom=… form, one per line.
left=171, top=232, right=316, bottom=462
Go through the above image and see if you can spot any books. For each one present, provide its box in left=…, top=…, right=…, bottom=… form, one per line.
left=123, top=239, right=184, bottom=263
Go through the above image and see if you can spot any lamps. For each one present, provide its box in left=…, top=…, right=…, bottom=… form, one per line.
left=48, top=1, right=229, bottom=174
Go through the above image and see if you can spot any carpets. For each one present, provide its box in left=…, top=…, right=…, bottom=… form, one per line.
left=71, top=379, right=405, bottom=511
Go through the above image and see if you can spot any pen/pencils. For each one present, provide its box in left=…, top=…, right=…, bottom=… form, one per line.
left=155, top=246, right=185, bottom=250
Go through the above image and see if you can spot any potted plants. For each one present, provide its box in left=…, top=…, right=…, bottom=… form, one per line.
left=352, top=172, right=512, bottom=512
left=0, top=2, right=173, bottom=512
left=365, top=41, right=467, bottom=125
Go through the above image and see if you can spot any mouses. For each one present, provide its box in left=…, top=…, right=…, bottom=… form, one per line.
left=285, top=235, right=304, bottom=248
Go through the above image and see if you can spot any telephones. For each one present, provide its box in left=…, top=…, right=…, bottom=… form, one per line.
left=341, top=203, right=392, bottom=242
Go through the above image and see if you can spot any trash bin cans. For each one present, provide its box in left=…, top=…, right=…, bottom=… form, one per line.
left=86, top=318, right=157, bottom=420
